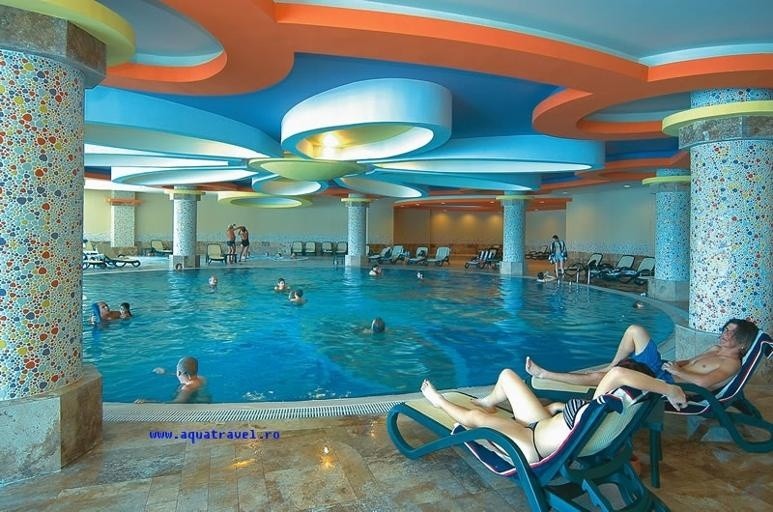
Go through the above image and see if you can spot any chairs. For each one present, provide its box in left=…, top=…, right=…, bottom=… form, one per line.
left=386, top=379, right=673, bottom=512
left=526, top=329, right=773, bottom=453
left=151, top=240, right=172, bottom=259
left=205, top=243, right=227, bottom=265
left=81, top=239, right=141, bottom=270
left=290, top=241, right=655, bottom=285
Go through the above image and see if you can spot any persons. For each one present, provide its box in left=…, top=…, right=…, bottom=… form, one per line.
left=416, top=271, right=425, bottom=281
left=134, top=355, right=205, bottom=405
left=368, top=263, right=381, bottom=276
left=548, top=235, right=568, bottom=277
left=288, top=289, right=305, bottom=303
left=633, top=300, right=646, bottom=310
left=91, top=301, right=121, bottom=324
left=526, top=317, right=759, bottom=400
left=420, top=359, right=686, bottom=465
left=119, top=302, right=130, bottom=317
left=363, top=318, right=386, bottom=332
left=274, top=278, right=286, bottom=290
left=536, top=270, right=556, bottom=283
left=225, top=223, right=297, bottom=263
left=208, top=276, right=218, bottom=287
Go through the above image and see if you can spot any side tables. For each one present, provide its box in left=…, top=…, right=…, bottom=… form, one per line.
left=141, top=247, right=156, bottom=256
left=224, top=254, right=238, bottom=263
